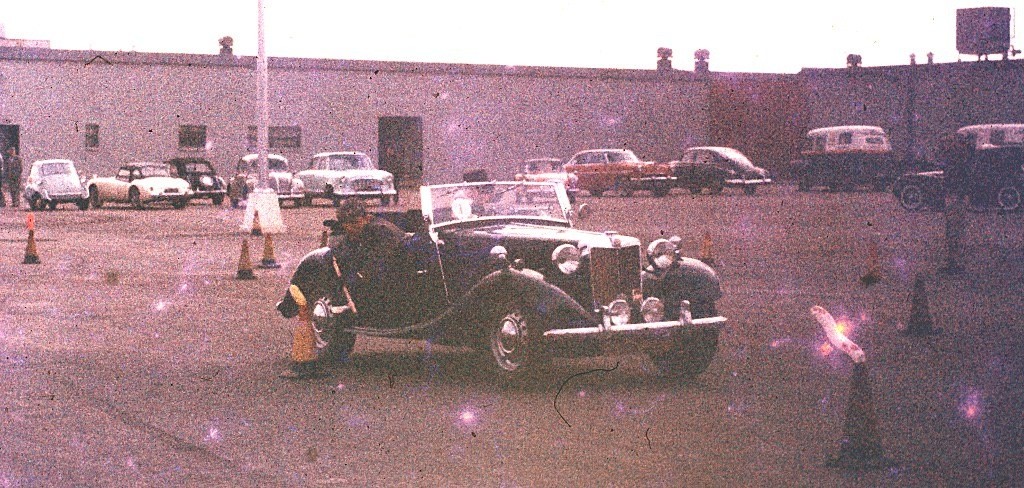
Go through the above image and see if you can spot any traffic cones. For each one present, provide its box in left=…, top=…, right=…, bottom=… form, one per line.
left=928, top=217, right=968, bottom=278
left=896, top=269, right=945, bottom=337
left=258, top=231, right=284, bottom=268
left=251, top=210, right=265, bottom=237
left=280, top=299, right=334, bottom=380
left=822, top=357, right=899, bottom=469
left=235, top=238, right=260, bottom=280
left=21, top=229, right=41, bottom=266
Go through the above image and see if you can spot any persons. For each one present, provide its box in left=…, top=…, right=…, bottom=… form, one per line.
left=0, top=144, right=23, bottom=207
left=334, top=195, right=406, bottom=317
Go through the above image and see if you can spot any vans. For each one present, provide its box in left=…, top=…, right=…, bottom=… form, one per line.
left=789, top=125, right=898, bottom=192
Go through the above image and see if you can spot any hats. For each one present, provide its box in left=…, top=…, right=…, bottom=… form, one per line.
left=462, top=169, right=494, bottom=193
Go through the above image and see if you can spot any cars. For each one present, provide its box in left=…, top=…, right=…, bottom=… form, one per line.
left=662, top=144, right=771, bottom=196
left=167, top=156, right=228, bottom=206
left=85, top=162, right=195, bottom=211
left=558, top=149, right=676, bottom=197
left=515, top=155, right=580, bottom=203
left=276, top=180, right=730, bottom=389
left=887, top=122, right=1024, bottom=214
left=225, top=152, right=307, bottom=209
left=296, top=151, right=399, bottom=208
left=22, top=158, right=90, bottom=212
